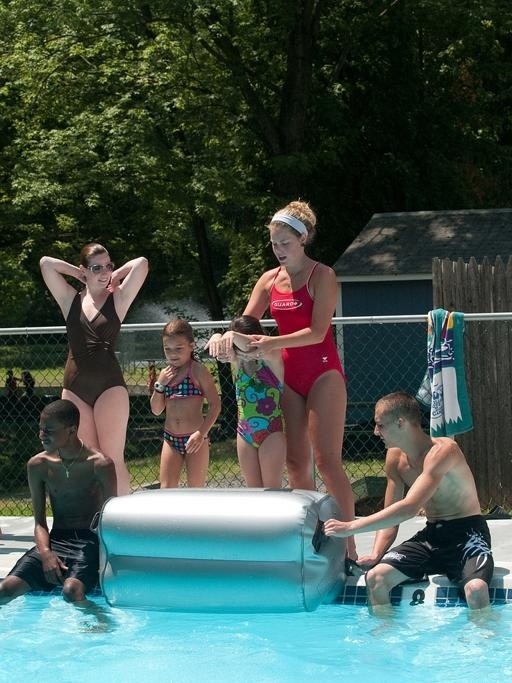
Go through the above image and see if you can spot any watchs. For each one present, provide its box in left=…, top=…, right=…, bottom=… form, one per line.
left=153, top=382, right=164, bottom=391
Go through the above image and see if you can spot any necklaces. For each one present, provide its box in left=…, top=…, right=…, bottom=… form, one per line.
left=58, top=439, right=84, bottom=479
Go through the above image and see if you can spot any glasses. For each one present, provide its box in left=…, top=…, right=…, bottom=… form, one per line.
left=86, top=263, right=114, bottom=273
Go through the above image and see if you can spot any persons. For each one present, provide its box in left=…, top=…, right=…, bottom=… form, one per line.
left=38, top=242, right=149, bottom=496
left=204, top=315, right=288, bottom=488
left=321, top=392, right=494, bottom=608
left=150, top=319, right=222, bottom=489
left=0, top=399, right=117, bottom=606
left=216, top=201, right=358, bottom=560
left=5, top=369, right=35, bottom=399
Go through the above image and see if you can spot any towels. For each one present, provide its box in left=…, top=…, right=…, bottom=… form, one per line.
left=415, top=308, right=475, bottom=439
